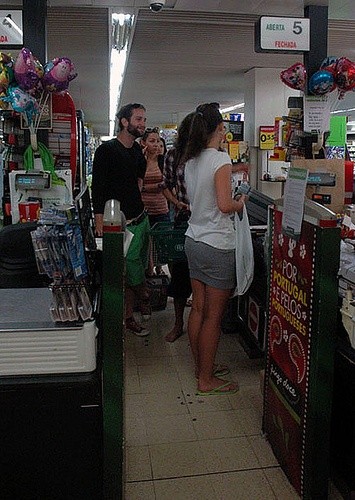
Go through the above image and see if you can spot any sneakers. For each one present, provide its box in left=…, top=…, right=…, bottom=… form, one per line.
left=139, top=296, right=152, bottom=321
left=124, top=318, right=150, bottom=336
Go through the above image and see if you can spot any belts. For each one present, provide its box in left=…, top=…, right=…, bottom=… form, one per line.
left=126, top=210, right=148, bottom=226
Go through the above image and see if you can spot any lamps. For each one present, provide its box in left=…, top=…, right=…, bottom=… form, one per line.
left=107, top=6, right=139, bottom=137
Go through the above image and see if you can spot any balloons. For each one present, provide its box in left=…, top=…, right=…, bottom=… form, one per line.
left=280, top=62, right=310, bottom=95
left=319, top=55, right=345, bottom=88
left=334, top=57, right=355, bottom=100
left=0, top=47, right=80, bottom=127
left=308, top=71, right=335, bottom=97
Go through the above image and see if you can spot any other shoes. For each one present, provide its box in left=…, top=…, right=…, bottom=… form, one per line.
left=146, top=268, right=154, bottom=277
left=186, top=299, right=193, bottom=307
left=155, top=268, right=165, bottom=275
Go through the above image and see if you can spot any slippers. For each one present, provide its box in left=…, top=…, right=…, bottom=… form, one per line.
left=166, top=330, right=183, bottom=342
left=198, top=381, right=239, bottom=396
left=196, top=364, right=229, bottom=381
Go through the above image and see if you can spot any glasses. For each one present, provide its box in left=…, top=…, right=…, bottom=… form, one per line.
left=145, top=126, right=158, bottom=133
left=197, top=102, right=220, bottom=112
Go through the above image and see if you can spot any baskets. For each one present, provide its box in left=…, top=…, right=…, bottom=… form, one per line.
left=132, top=273, right=171, bottom=312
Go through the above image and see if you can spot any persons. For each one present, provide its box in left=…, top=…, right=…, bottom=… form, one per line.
left=135, top=124, right=191, bottom=281
left=156, top=137, right=170, bottom=171
left=186, top=100, right=250, bottom=396
left=89, top=102, right=161, bottom=337
left=163, top=109, right=205, bottom=343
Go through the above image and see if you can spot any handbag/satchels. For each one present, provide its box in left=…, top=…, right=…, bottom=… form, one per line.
left=234, top=199, right=254, bottom=295
left=9, top=142, right=72, bottom=225
